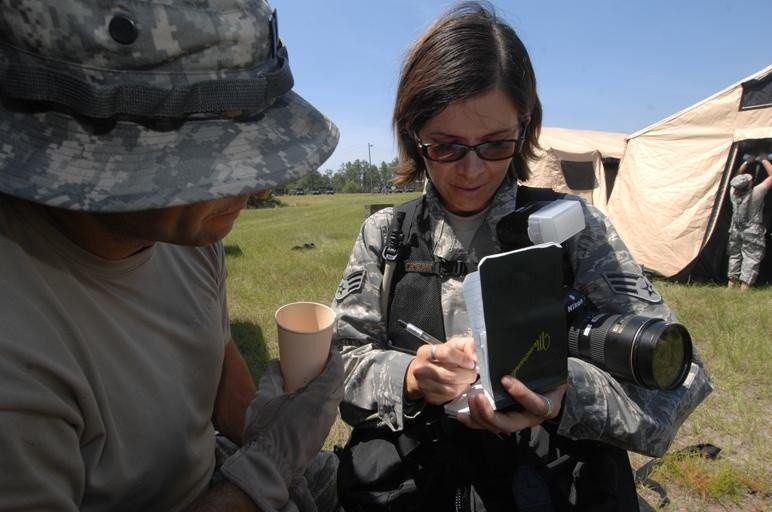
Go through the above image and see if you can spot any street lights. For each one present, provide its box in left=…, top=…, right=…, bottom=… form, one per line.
left=367, top=142, right=376, bottom=194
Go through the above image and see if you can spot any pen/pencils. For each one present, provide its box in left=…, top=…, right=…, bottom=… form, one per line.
left=396, top=319, right=482, bottom=375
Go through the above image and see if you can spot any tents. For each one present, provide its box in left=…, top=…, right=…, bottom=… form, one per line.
left=515, top=124, right=629, bottom=225
left=599, top=64, right=771, bottom=282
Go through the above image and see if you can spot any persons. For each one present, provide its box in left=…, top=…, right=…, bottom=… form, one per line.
left=2, top=2, right=359, bottom=509
left=725, top=149, right=772, bottom=293
left=326, top=0, right=713, bottom=509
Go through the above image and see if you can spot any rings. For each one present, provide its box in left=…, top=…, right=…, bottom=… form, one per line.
left=429, top=343, right=440, bottom=362
left=542, top=395, right=554, bottom=420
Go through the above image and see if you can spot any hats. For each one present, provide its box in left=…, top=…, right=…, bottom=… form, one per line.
left=730, top=173, right=752, bottom=190
left=0, top=1, right=340, bottom=213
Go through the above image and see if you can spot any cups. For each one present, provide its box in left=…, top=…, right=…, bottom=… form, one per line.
left=274, top=300, right=339, bottom=392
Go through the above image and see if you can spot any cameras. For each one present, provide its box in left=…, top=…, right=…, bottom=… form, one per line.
left=497, top=198, right=693, bottom=394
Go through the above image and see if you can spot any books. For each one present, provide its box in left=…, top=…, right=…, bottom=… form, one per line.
left=441, top=241, right=569, bottom=421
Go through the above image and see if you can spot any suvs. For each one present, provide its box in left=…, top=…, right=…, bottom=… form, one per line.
left=373, top=185, right=415, bottom=194
left=311, top=187, right=321, bottom=194
left=325, top=185, right=334, bottom=194
left=295, top=186, right=306, bottom=195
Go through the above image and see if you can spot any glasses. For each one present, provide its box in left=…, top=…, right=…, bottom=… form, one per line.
left=410, top=130, right=525, bottom=163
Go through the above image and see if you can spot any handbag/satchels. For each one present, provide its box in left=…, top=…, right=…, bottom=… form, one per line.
left=336, top=405, right=473, bottom=509
